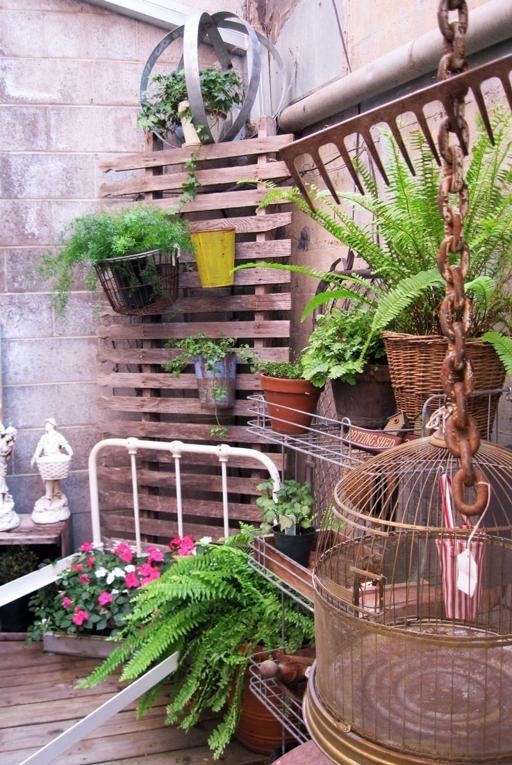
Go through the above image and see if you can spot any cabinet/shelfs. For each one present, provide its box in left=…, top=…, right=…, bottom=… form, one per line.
left=246, top=391, right=456, bottom=744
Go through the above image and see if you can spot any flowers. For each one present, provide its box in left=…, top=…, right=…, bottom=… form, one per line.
left=25, top=535, right=206, bottom=645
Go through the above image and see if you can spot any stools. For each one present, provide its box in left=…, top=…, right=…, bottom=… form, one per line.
left=0, top=513, right=72, bottom=557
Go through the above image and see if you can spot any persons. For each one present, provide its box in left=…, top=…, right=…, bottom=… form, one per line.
left=28, top=418, right=74, bottom=498
left=1, top=425, right=18, bottom=501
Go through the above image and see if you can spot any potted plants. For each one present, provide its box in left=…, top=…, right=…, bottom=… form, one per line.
left=240, top=344, right=326, bottom=434
left=299, top=310, right=395, bottom=429
left=255, top=479, right=316, bottom=571
left=137, top=71, right=243, bottom=204
left=229, top=106, right=512, bottom=440
left=161, top=331, right=236, bottom=437
left=73, top=521, right=312, bottom=761
left=38, top=205, right=197, bottom=317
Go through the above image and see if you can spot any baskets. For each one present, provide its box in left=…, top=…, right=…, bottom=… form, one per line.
left=89, top=240, right=183, bottom=319
left=375, top=320, right=512, bottom=453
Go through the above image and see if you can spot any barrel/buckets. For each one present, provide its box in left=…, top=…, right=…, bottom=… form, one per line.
left=193, top=350, right=237, bottom=411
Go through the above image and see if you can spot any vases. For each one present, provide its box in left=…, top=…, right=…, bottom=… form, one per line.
left=41, top=629, right=115, bottom=657
